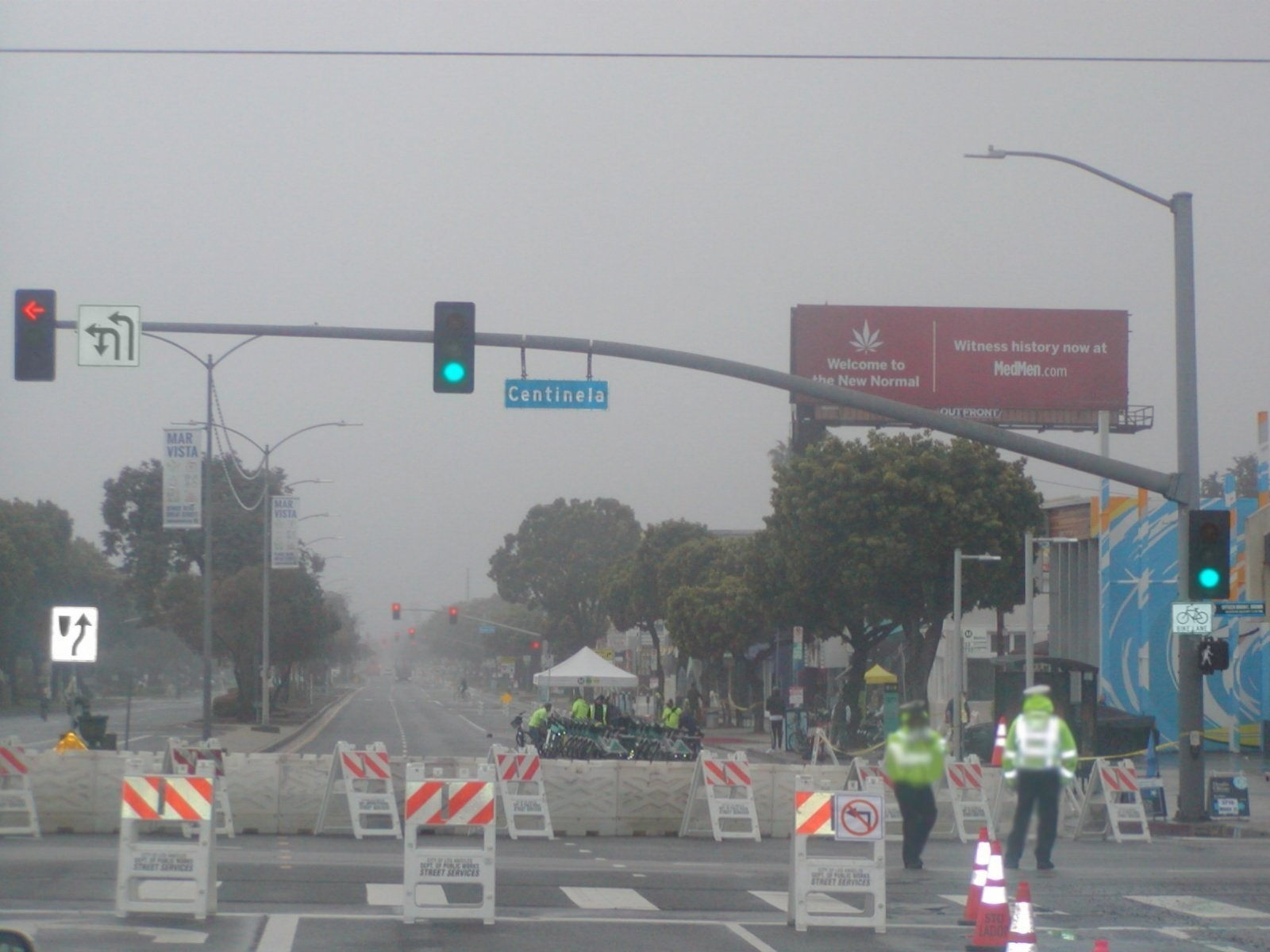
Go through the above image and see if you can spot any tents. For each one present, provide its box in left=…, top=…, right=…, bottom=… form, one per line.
left=532, top=645, right=641, bottom=690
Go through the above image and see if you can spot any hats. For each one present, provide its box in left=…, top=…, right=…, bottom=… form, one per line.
left=1023, top=685, right=1052, bottom=697
left=899, top=699, right=926, bottom=711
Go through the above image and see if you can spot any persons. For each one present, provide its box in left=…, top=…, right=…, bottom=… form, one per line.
left=882, top=698, right=951, bottom=873
left=943, top=692, right=973, bottom=758
left=0, top=672, right=884, bottom=765
left=999, top=681, right=1079, bottom=872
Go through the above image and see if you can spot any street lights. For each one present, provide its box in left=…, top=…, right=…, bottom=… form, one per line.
left=969, top=141, right=1216, bottom=824
left=141, top=327, right=347, bottom=740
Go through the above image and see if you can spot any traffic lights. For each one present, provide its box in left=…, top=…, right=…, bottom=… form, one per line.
left=430, top=299, right=479, bottom=391
left=10, top=288, right=56, bottom=382
left=1191, top=510, right=1230, bottom=678
left=391, top=602, right=541, bottom=652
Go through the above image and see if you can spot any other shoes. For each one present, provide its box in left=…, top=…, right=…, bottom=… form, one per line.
left=765, top=749, right=784, bottom=754
left=1037, top=860, right=1055, bottom=869
left=1003, top=859, right=1019, bottom=868
left=905, top=857, right=924, bottom=870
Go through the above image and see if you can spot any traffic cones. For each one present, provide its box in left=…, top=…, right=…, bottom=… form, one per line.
left=964, top=825, right=1114, bottom=952
left=0, top=739, right=1153, bottom=932
